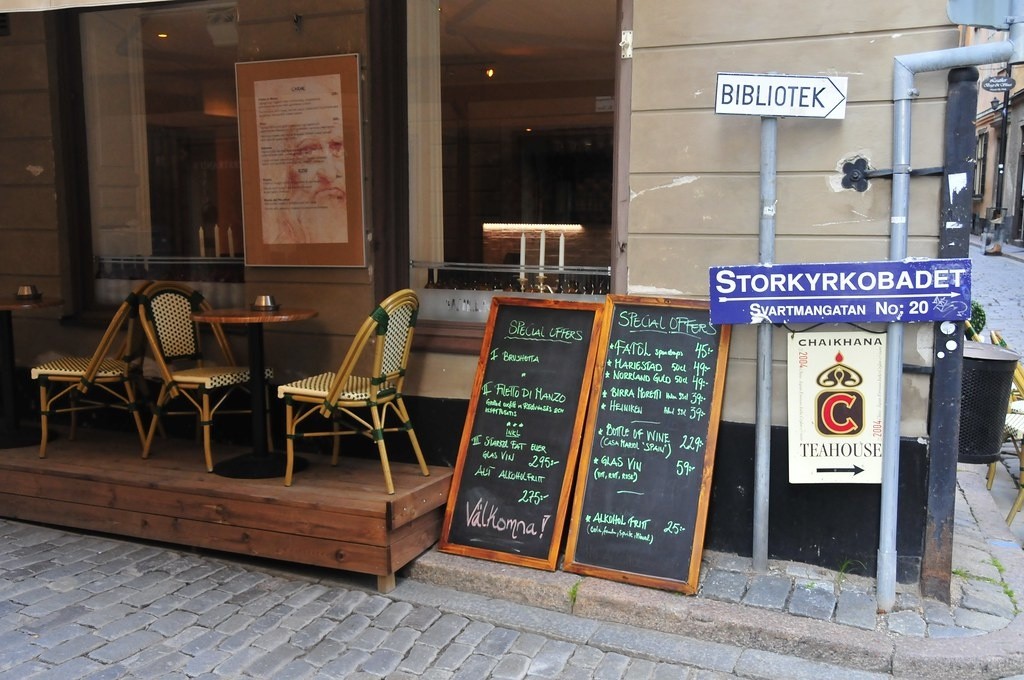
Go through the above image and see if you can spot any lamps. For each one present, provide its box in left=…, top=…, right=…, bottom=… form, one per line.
left=991, top=96, right=1005, bottom=111
left=204, top=4, right=241, bottom=48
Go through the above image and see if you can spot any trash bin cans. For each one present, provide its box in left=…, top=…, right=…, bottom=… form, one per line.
left=956, top=340, right=1019, bottom=463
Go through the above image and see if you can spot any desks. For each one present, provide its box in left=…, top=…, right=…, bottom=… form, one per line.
left=0, top=296, right=65, bottom=448
left=189, top=308, right=319, bottom=479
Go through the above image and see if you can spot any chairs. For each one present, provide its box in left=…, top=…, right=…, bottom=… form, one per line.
left=276, top=288, right=430, bottom=495
left=963, top=319, right=1024, bottom=525
left=138, top=281, right=274, bottom=472
left=31, top=279, right=167, bottom=458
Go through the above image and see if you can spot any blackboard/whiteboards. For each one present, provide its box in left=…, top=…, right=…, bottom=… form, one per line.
left=559, top=295, right=731, bottom=598
left=437, top=296, right=606, bottom=575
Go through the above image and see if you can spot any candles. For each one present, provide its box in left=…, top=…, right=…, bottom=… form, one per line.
left=227, top=227, right=234, bottom=257
left=519, top=232, right=526, bottom=279
left=214, top=224, right=220, bottom=257
left=539, top=229, right=545, bottom=277
left=199, top=226, right=205, bottom=257
left=559, top=233, right=565, bottom=271
